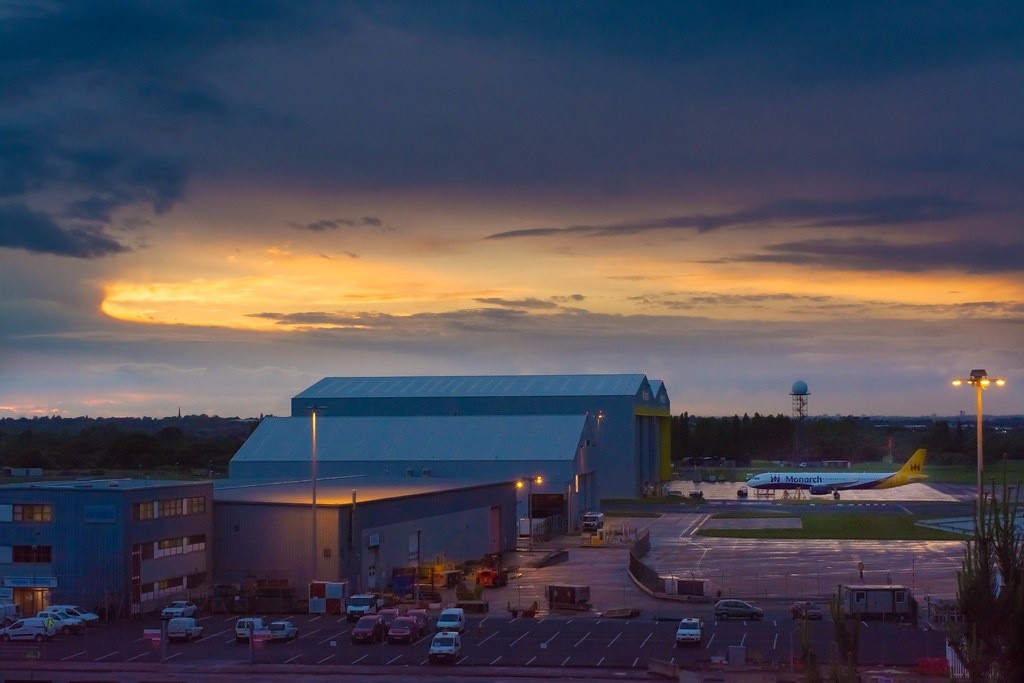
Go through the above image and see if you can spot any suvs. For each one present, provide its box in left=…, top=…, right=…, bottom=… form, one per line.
left=268, top=621, right=299, bottom=643
left=166, top=618, right=204, bottom=643
left=715, top=600, right=764, bottom=621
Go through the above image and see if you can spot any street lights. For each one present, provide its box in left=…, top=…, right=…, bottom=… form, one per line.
left=953, top=369, right=1006, bottom=575
left=515, top=475, right=543, bottom=547
left=304, top=400, right=328, bottom=581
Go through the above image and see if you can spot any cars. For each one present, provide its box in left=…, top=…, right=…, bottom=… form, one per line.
left=160, top=601, right=198, bottom=620
left=26, top=611, right=83, bottom=635
left=351, top=615, right=389, bottom=646
left=388, top=617, right=419, bottom=645
left=377, top=608, right=400, bottom=628
left=405, top=608, right=427, bottom=635
left=676, top=618, right=703, bottom=643
left=790, top=601, right=824, bottom=620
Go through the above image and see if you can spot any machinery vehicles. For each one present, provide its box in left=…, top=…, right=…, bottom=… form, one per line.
left=475, top=553, right=508, bottom=588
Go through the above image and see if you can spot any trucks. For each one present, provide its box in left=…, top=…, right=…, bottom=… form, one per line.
left=582, top=512, right=604, bottom=531
left=346, top=595, right=384, bottom=622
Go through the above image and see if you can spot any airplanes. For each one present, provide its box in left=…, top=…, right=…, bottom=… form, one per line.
left=747, top=448, right=930, bottom=498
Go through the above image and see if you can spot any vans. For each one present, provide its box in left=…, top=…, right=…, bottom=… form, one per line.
left=429, top=632, right=462, bottom=664
left=235, top=618, right=268, bottom=643
left=45, top=606, right=99, bottom=627
left=436, top=608, right=466, bottom=635
left=0, top=618, right=56, bottom=643
